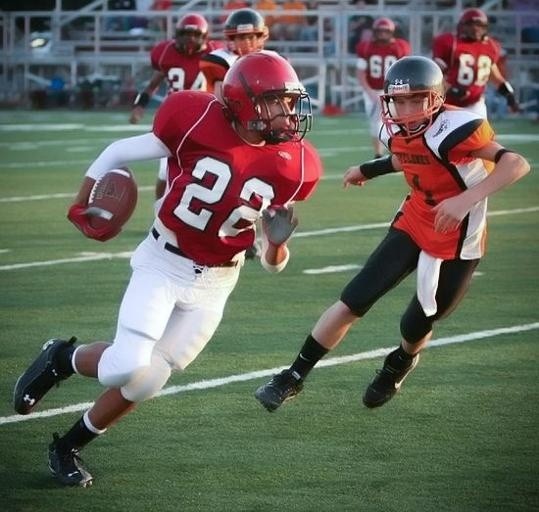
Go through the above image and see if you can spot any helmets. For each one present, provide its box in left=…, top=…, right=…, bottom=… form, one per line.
left=176, top=14, right=209, bottom=52
left=222, top=50, right=313, bottom=145
left=224, top=8, right=268, bottom=52
left=373, top=19, right=395, bottom=42
left=459, top=8, right=488, bottom=42
left=379, top=56, right=446, bottom=141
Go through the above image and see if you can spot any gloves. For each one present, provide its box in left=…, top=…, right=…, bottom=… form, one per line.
left=449, top=88, right=469, bottom=101
left=65, top=204, right=116, bottom=242
left=262, top=202, right=299, bottom=246
left=507, top=95, right=519, bottom=113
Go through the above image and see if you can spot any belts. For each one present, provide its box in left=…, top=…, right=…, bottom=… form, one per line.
left=151, top=226, right=238, bottom=267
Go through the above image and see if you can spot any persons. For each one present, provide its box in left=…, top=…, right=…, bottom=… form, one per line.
left=14, top=50, right=322, bottom=485
left=348, top=0, right=376, bottom=52
left=356, top=19, right=412, bottom=158
left=130, top=14, right=234, bottom=199
left=156, top=1, right=335, bottom=40
left=200, top=9, right=269, bottom=100
left=426, top=8, right=524, bottom=120
left=253, top=57, right=531, bottom=412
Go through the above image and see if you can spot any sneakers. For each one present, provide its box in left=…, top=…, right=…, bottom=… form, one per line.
left=47, top=442, right=94, bottom=487
left=13, top=337, right=78, bottom=414
left=364, top=349, right=419, bottom=408
left=255, top=369, right=304, bottom=412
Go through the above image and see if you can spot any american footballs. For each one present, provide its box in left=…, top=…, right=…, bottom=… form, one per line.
left=88, top=167, right=138, bottom=229
